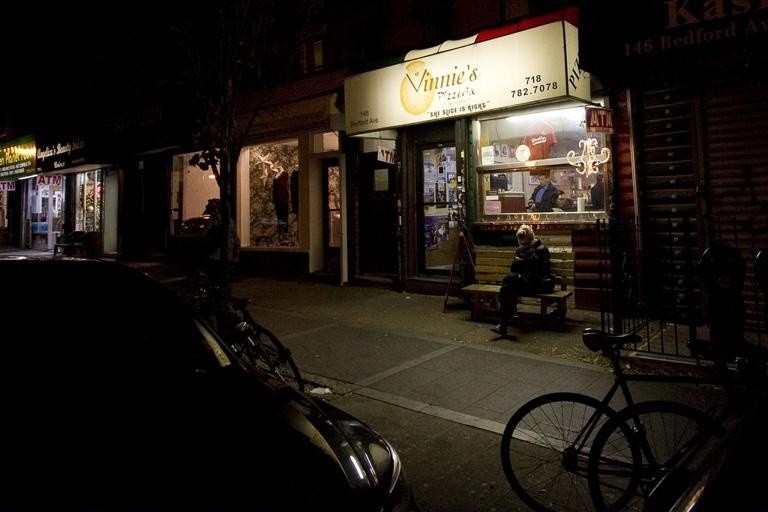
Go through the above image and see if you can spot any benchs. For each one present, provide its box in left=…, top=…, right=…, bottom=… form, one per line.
left=54, top=234, right=86, bottom=258
left=460, top=250, right=576, bottom=331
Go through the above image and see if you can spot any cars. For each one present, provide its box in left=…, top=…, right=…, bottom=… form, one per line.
left=1, top=250, right=421, bottom=512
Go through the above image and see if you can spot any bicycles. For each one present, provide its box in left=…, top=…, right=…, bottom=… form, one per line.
left=501, top=328, right=767, bottom=511
left=191, top=277, right=304, bottom=393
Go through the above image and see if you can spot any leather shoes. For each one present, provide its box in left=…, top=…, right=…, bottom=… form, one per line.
left=490, top=323, right=508, bottom=335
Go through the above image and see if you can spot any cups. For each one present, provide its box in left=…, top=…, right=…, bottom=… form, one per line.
left=576, top=197, right=586, bottom=212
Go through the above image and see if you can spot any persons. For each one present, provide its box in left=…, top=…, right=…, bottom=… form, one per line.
left=526, top=171, right=559, bottom=213
left=490, top=221, right=551, bottom=337
left=271, top=164, right=290, bottom=236
left=288, top=163, right=299, bottom=217
left=590, top=172, right=604, bottom=212
